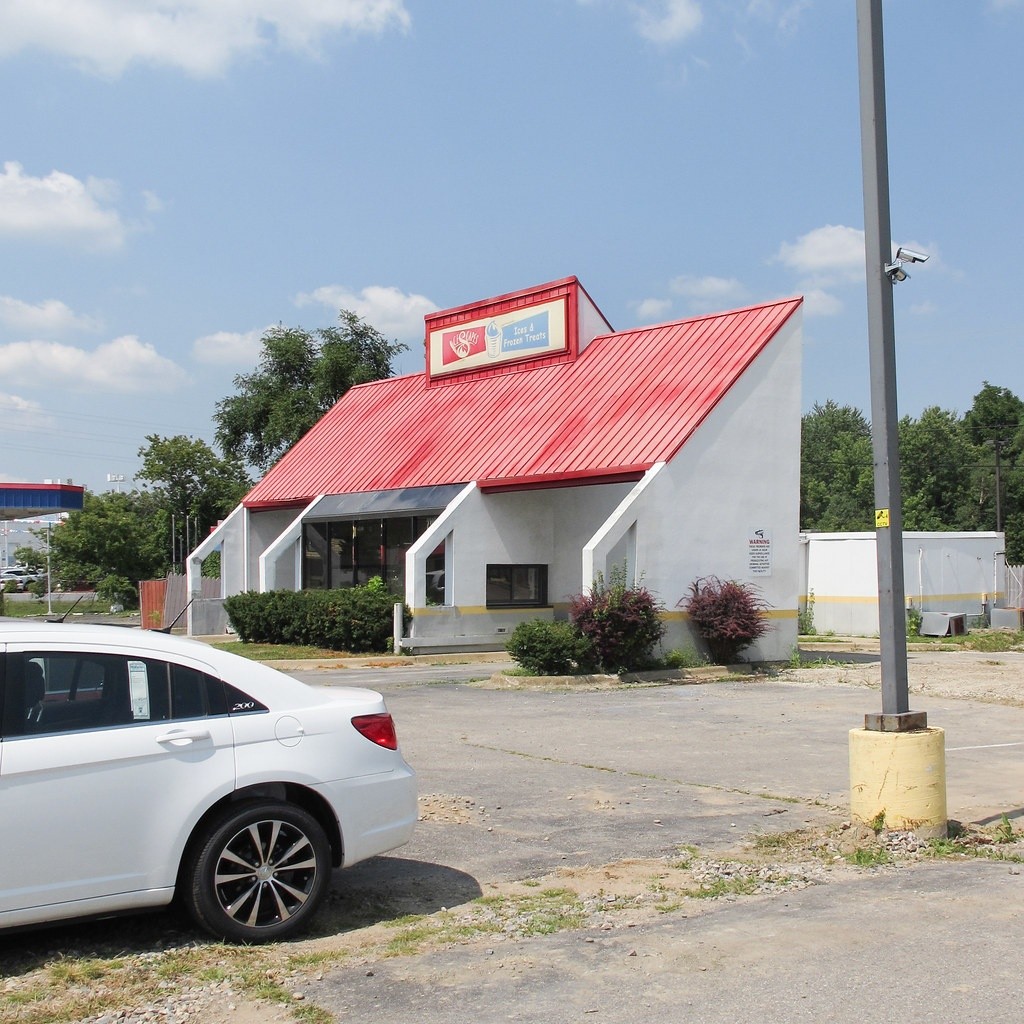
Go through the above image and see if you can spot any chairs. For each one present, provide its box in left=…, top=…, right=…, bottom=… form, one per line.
left=20, top=661, right=46, bottom=735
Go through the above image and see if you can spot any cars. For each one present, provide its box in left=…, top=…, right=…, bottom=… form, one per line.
left=0, top=620, right=419, bottom=943
left=29, top=657, right=104, bottom=704
left=0, top=566, right=47, bottom=594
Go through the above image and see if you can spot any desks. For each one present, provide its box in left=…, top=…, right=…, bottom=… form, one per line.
left=919, top=612, right=968, bottom=637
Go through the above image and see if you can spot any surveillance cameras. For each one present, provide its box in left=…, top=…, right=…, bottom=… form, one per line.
left=890, top=266, right=912, bottom=282
left=895, top=247, right=930, bottom=264
